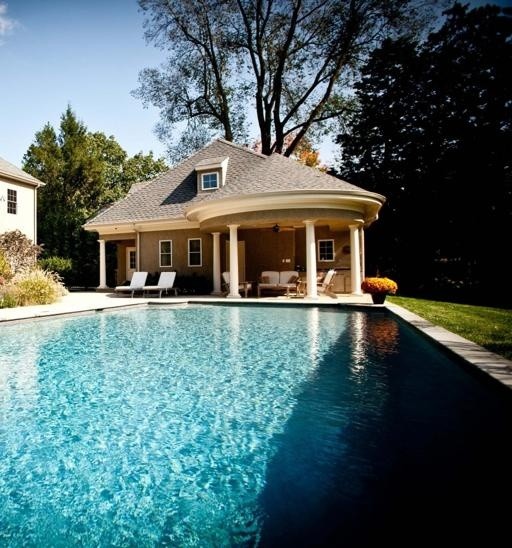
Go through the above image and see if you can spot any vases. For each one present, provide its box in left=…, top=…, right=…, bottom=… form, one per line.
left=371, top=293, right=387, bottom=304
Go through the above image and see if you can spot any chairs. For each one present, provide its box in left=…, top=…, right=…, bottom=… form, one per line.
left=114, top=269, right=337, bottom=299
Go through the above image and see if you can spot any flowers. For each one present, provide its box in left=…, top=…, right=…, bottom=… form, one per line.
left=361, top=276, right=399, bottom=295
